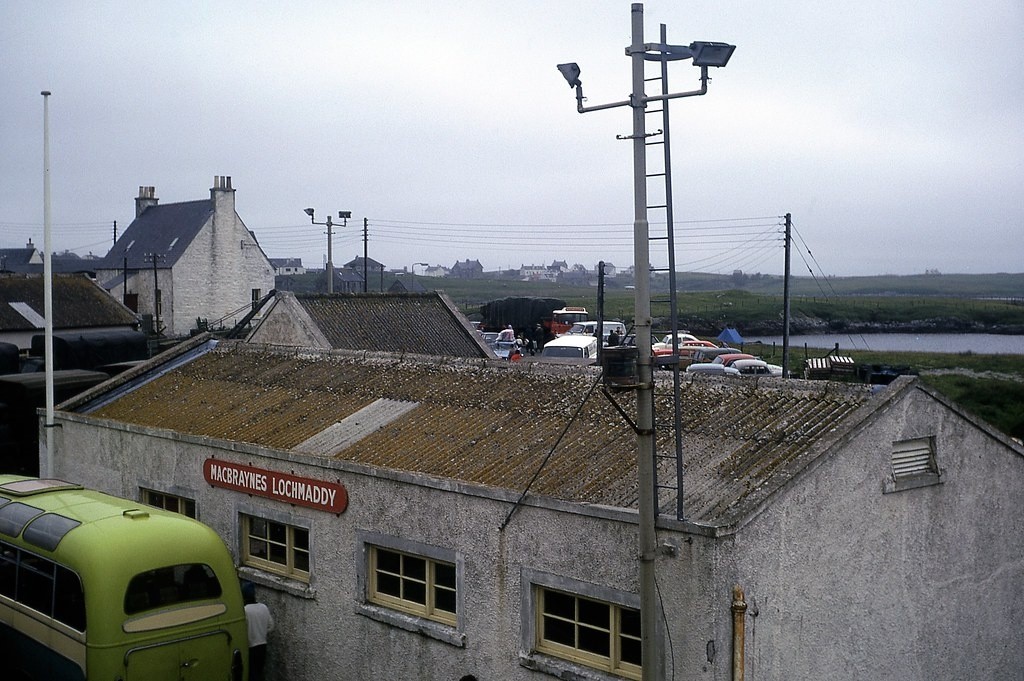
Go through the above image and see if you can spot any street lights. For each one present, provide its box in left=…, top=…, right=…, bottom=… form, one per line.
left=412, top=262, right=429, bottom=291
left=305, top=207, right=352, bottom=293
left=556, top=38, right=737, bottom=680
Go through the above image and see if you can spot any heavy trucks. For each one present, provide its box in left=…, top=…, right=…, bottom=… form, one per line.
left=475, top=296, right=589, bottom=336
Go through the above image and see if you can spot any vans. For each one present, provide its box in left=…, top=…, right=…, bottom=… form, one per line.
left=564, top=320, right=627, bottom=345
left=541, top=335, right=598, bottom=360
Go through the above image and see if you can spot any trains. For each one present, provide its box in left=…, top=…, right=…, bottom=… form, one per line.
left=0, top=471, right=251, bottom=681
left=0, top=359, right=142, bottom=445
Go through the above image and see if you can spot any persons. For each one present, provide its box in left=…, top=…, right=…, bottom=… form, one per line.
left=608, top=330, right=619, bottom=346
left=718, top=340, right=729, bottom=348
left=511, top=350, right=521, bottom=360
left=615, top=327, right=623, bottom=335
left=509, top=344, right=516, bottom=359
left=517, top=323, right=554, bottom=357
left=501, top=323, right=512, bottom=330
left=593, top=329, right=597, bottom=337
left=583, top=329, right=589, bottom=335
left=240, top=580, right=274, bottom=681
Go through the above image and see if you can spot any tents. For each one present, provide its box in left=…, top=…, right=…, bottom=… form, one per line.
left=716, top=328, right=744, bottom=345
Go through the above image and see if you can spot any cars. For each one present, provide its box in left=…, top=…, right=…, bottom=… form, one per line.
left=651, top=331, right=792, bottom=379
